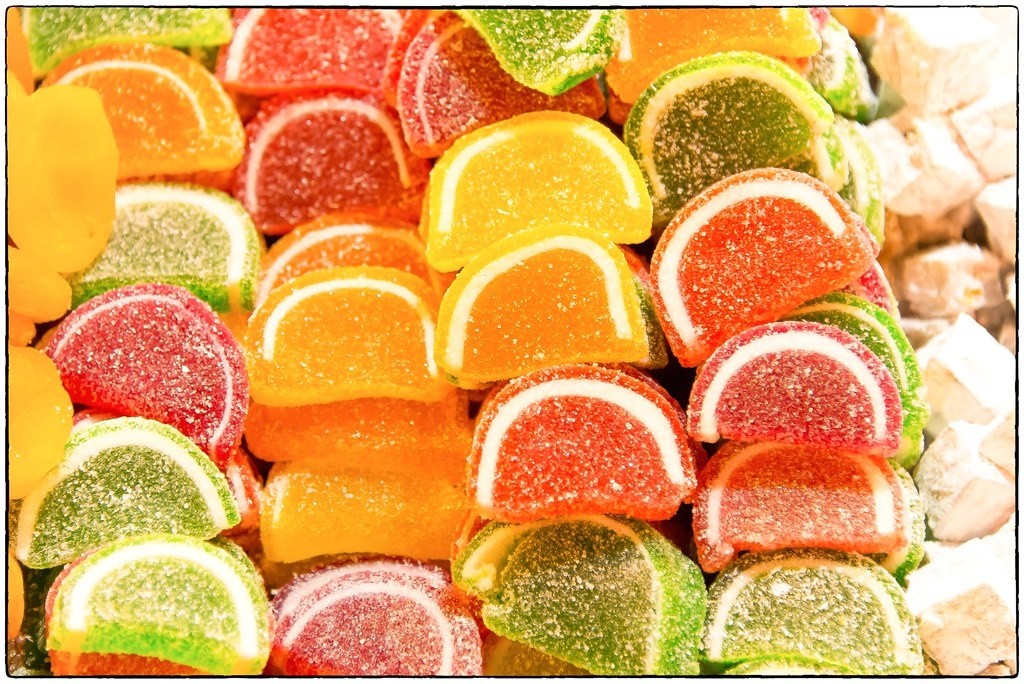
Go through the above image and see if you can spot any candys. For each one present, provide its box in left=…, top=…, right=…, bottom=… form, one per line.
left=6, top=8, right=928, bottom=677
